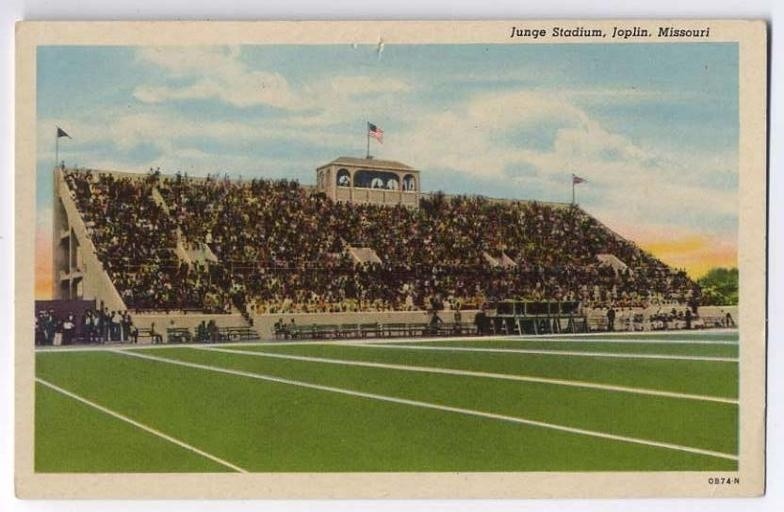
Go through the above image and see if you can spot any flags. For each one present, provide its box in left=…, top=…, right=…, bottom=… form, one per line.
left=57, top=126, right=71, bottom=139
left=368, top=123, right=384, bottom=144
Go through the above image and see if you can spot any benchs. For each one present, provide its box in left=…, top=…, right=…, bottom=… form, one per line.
left=703, top=315, right=728, bottom=328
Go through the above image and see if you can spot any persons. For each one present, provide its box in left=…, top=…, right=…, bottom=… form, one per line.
left=34, top=163, right=739, bottom=347
